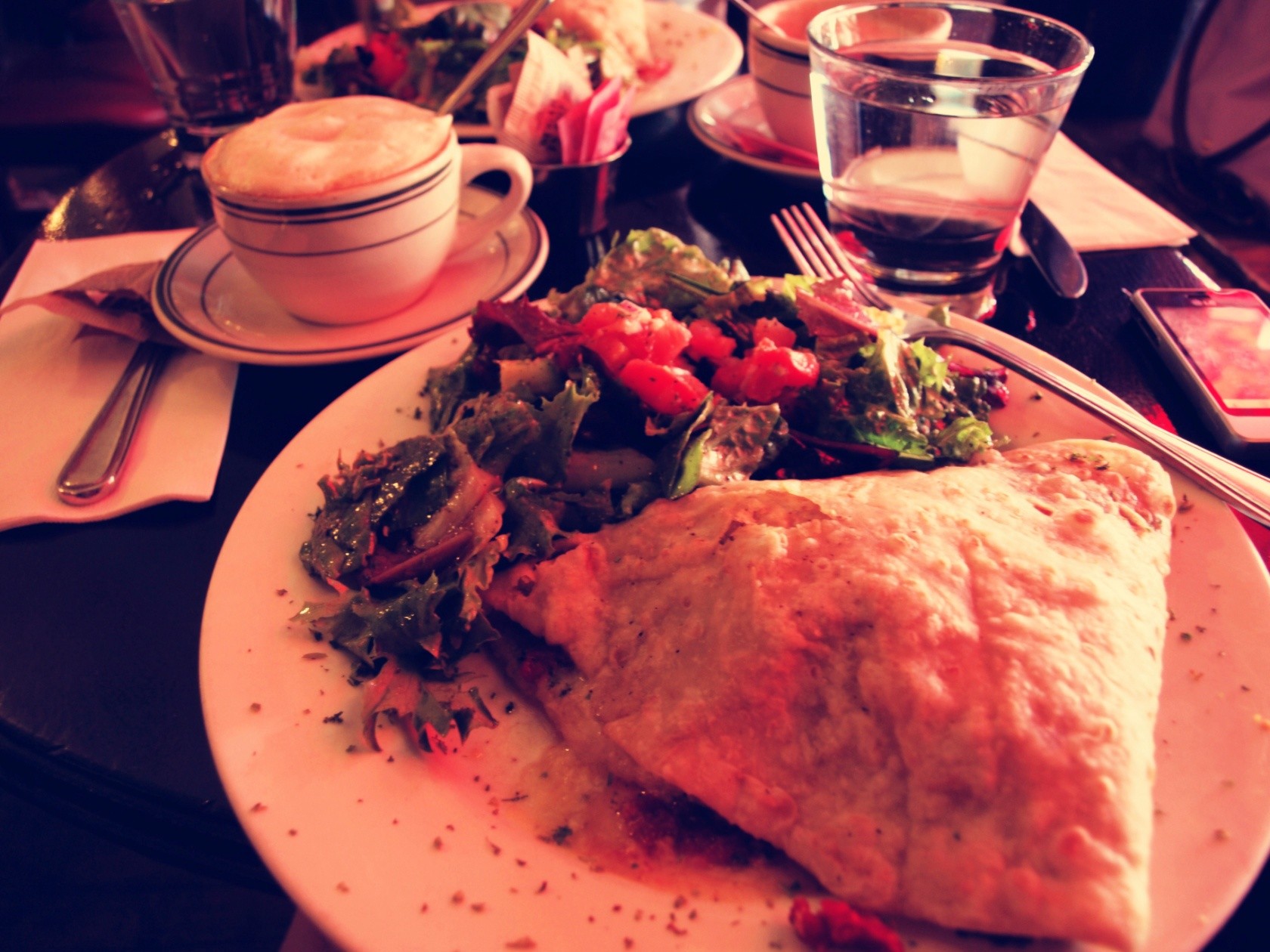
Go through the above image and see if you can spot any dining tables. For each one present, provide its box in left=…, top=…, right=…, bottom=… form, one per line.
left=0, top=67, right=1270, bottom=906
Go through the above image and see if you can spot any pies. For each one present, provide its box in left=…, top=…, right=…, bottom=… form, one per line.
left=479, top=440, right=1178, bottom=952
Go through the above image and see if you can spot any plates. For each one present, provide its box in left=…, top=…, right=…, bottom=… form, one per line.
left=694, top=79, right=822, bottom=183
left=195, top=259, right=1270, bottom=949
left=294, top=8, right=747, bottom=140
left=150, top=185, right=552, bottom=372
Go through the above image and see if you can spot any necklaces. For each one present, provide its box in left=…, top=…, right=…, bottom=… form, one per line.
left=1172, top=3, right=1270, bottom=168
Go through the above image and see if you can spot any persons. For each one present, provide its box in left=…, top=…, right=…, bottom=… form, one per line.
left=1079, top=1, right=1270, bottom=242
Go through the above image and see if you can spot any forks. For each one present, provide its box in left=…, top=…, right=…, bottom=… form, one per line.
left=761, top=195, right=1270, bottom=551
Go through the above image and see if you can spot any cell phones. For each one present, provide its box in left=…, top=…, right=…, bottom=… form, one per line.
left=1133, top=284, right=1269, bottom=452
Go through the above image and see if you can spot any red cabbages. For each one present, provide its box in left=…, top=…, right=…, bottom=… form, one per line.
left=289, top=228, right=1012, bottom=757
left=299, top=0, right=605, bottom=125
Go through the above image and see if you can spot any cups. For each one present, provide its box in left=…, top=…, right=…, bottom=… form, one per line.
left=106, top=0, right=302, bottom=169
left=200, top=104, right=535, bottom=329
left=803, top=0, right=1098, bottom=324
left=742, top=2, right=949, bottom=155
